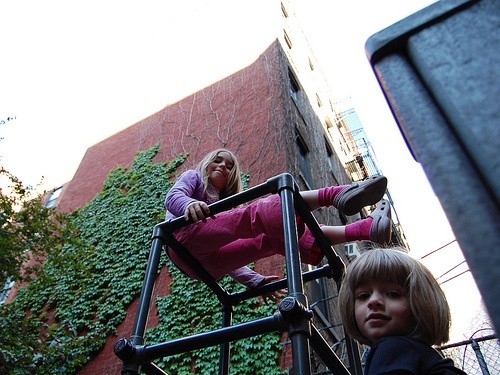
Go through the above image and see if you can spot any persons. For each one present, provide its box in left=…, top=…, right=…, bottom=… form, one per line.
left=163, top=148, right=394, bottom=307
left=339, top=247, right=470, bottom=375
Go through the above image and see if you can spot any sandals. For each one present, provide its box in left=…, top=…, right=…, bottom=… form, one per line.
left=367, top=199, right=392, bottom=245
left=332, top=174, right=387, bottom=216
